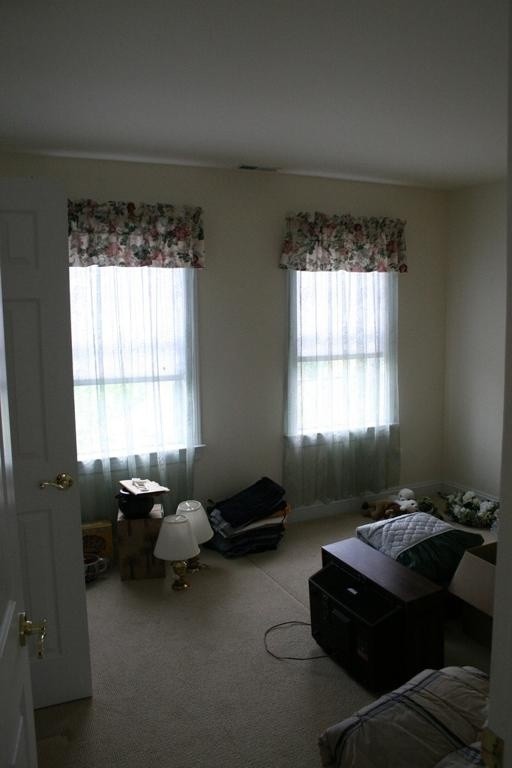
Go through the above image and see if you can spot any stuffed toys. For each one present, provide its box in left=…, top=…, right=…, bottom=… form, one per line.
left=361, top=488, right=419, bottom=521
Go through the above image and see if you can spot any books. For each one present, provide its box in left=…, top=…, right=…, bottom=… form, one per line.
left=119, top=477, right=170, bottom=496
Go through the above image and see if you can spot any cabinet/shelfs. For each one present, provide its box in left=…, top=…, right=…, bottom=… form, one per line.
left=307, top=534, right=449, bottom=697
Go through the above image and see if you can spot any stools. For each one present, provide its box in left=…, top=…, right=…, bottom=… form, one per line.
left=356, top=510, right=484, bottom=585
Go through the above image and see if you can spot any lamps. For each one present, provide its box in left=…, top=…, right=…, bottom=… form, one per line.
left=154, top=514, right=200, bottom=592
left=176, top=500, right=215, bottom=573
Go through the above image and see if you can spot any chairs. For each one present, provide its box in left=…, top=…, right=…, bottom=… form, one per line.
left=319, top=663, right=503, bottom=767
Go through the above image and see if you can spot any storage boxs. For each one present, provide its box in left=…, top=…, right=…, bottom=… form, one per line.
left=116, top=505, right=166, bottom=581
left=81, top=520, right=114, bottom=565
left=448, top=541, right=497, bottom=650
